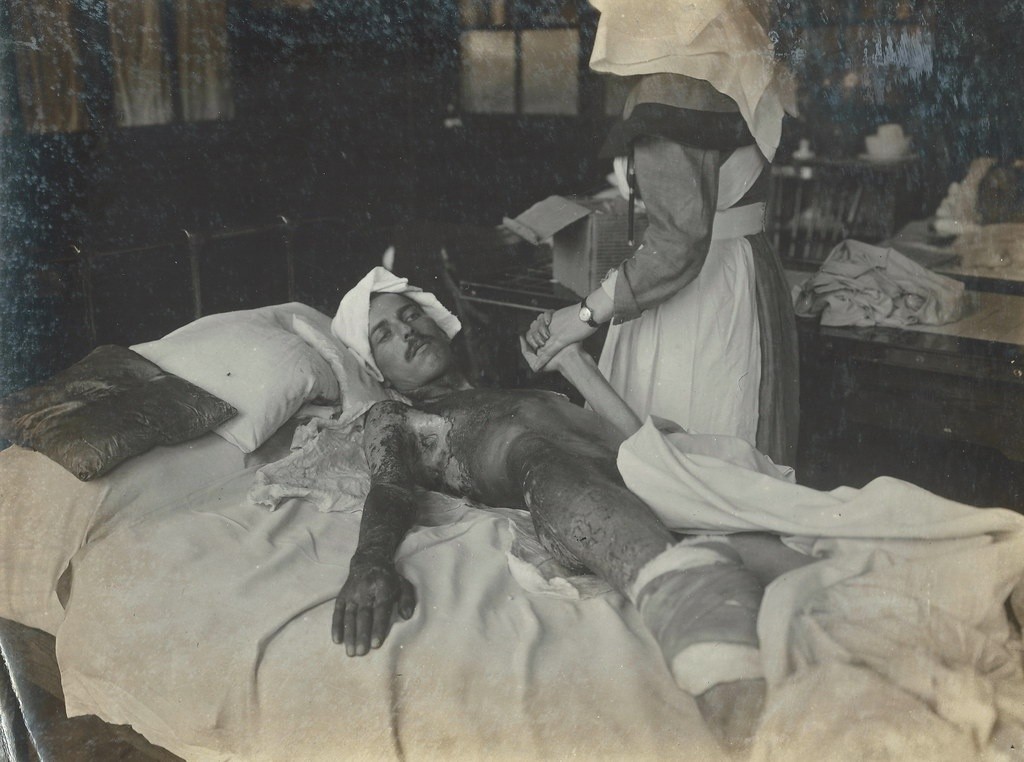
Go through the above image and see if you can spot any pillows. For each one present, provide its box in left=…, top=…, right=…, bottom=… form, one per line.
left=126, top=300, right=355, bottom=457
left=0, top=342, right=240, bottom=483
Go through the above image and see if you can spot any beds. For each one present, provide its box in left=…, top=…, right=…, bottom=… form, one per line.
left=3, top=398, right=1024, bottom=762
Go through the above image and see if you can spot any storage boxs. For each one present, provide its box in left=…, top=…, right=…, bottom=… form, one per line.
left=500, top=194, right=650, bottom=299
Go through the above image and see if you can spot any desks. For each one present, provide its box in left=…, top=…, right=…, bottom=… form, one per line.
left=818, top=281, right=1024, bottom=477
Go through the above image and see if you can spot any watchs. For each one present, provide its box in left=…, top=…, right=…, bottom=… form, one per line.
left=578, top=296, right=602, bottom=327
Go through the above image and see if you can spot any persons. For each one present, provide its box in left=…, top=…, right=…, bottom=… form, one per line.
left=523, top=0, right=802, bottom=483
left=334, top=291, right=1024, bottom=754
